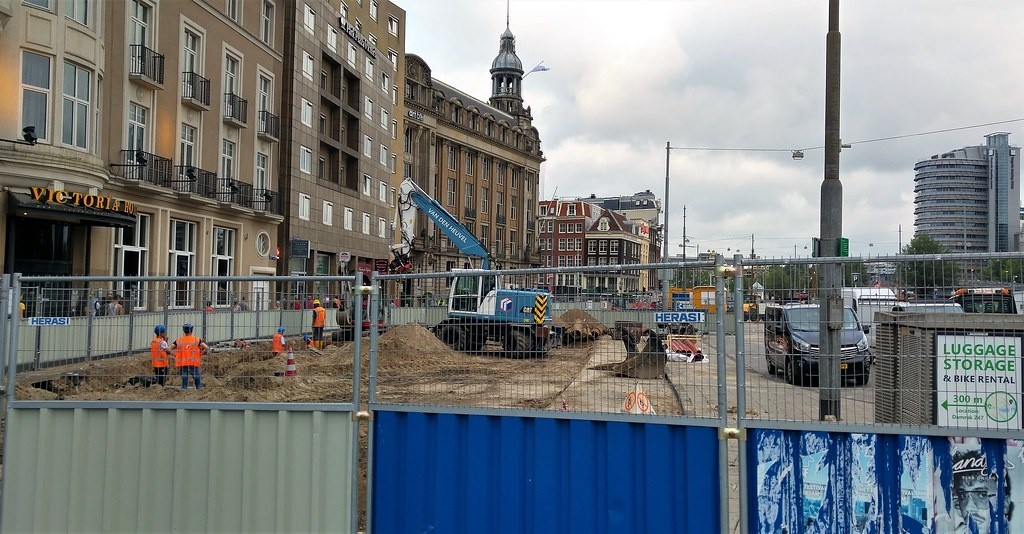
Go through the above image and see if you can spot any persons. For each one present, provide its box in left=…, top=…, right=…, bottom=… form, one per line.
left=18, top=292, right=444, bottom=390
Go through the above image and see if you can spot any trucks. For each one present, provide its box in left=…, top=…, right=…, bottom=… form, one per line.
left=841, top=287, right=897, bottom=333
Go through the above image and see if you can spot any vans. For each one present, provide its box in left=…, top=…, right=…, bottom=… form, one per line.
left=764, top=303, right=872, bottom=385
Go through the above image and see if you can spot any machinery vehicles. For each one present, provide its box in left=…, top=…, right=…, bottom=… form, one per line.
left=331, top=286, right=389, bottom=342
left=742, top=294, right=765, bottom=319
left=386, top=177, right=554, bottom=359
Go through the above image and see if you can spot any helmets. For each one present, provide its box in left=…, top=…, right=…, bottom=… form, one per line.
left=183, top=323, right=194, bottom=331
left=277, top=328, right=285, bottom=333
left=155, top=325, right=167, bottom=333
left=313, top=300, right=320, bottom=304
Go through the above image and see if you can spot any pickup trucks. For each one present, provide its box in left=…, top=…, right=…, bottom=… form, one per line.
left=869, top=303, right=967, bottom=359
left=948, top=288, right=1020, bottom=313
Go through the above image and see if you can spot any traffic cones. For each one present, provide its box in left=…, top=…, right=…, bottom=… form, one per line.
left=285, top=345, right=304, bottom=378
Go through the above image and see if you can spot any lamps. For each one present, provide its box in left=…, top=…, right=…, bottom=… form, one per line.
left=0, top=124, right=38, bottom=147
left=110, top=152, right=149, bottom=170
left=209, top=181, right=239, bottom=196
left=164, top=169, right=197, bottom=184
left=246, top=192, right=276, bottom=204
